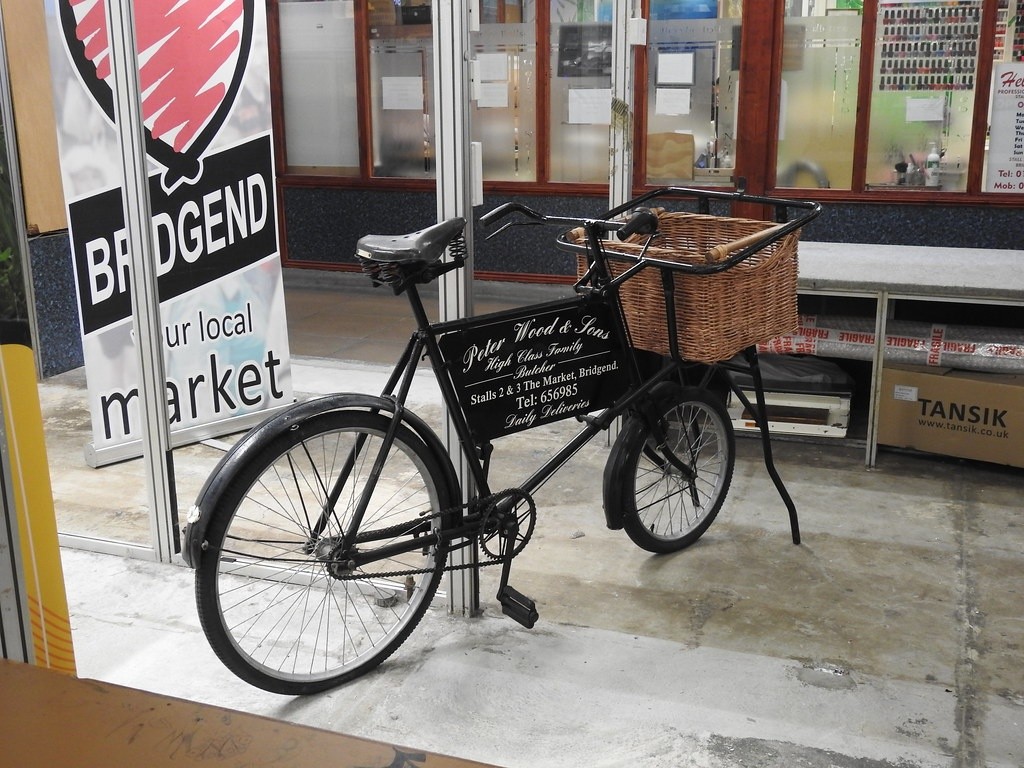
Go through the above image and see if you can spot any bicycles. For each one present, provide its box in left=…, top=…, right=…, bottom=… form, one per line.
left=182, top=182, right=826, bottom=697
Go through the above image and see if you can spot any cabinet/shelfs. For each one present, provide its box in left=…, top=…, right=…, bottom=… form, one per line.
left=664, top=238, right=1024, bottom=471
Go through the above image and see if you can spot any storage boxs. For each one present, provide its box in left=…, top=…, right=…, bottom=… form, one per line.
left=878, top=363, right=1024, bottom=469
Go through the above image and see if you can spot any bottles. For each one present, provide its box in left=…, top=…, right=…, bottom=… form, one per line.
left=892, top=141, right=944, bottom=188
left=709, top=150, right=731, bottom=168
left=878, top=0, right=1024, bottom=91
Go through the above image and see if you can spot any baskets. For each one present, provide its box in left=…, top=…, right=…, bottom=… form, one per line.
left=572, top=211, right=800, bottom=363
